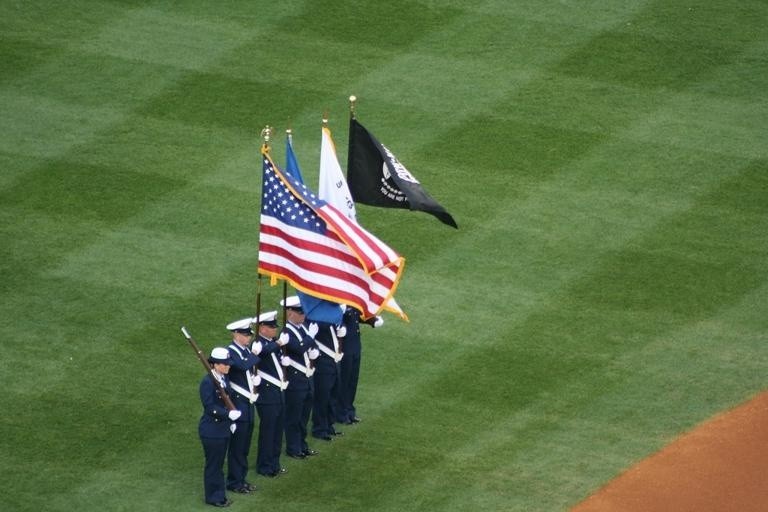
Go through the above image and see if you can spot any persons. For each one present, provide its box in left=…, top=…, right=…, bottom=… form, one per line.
left=198, top=296, right=384, bottom=508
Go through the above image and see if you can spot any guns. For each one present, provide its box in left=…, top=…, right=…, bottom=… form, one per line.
left=180, top=326, right=237, bottom=410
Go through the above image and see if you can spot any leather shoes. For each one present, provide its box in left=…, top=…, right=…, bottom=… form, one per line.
left=208, top=415, right=362, bottom=507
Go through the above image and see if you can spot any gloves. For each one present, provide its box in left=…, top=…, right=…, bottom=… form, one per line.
left=280, top=354, right=289, bottom=366
left=228, top=409, right=241, bottom=421
left=374, top=315, right=384, bottom=328
left=336, top=325, right=346, bottom=338
left=251, top=340, right=262, bottom=356
left=308, top=348, right=319, bottom=360
left=250, top=374, right=261, bottom=386
left=308, top=322, right=319, bottom=340
left=275, top=332, right=289, bottom=346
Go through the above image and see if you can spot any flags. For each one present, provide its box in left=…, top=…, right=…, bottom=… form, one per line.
left=257, top=118, right=410, bottom=329
left=346, top=117, right=459, bottom=230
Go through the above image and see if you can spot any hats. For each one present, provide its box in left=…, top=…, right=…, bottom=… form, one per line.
left=226, top=311, right=280, bottom=336
left=208, top=347, right=235, bottom=366
left=280, top=296, right=304, bottom=314
